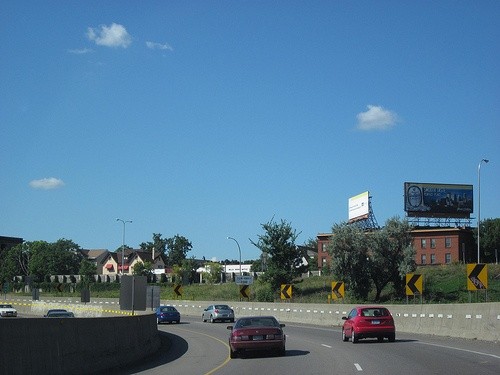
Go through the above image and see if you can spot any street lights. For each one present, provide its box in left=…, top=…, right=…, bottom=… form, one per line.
left=115, top=218, right=132, bottom=274
left=227, top=236, right=241, bottom=275
left=477, top=158, right=489, bottom=264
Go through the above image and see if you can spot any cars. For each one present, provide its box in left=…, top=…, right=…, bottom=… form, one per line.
left=202, top=305, right=234, bottom=323
left=226, top=315, right=287, bottom=359
left=153, top=306, right=180, bottom=324
left=341, top=305, right=395, bottom=344
left=43, top=309, right=75, bottom=318
left=0, top=304, right=17, bottom=318
